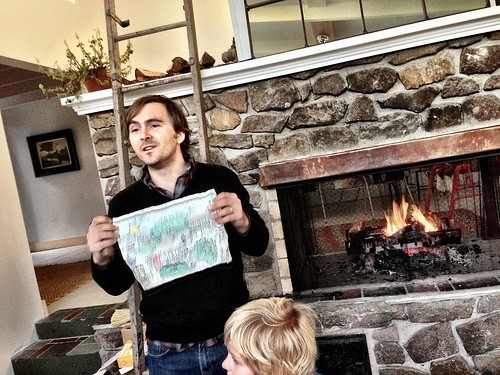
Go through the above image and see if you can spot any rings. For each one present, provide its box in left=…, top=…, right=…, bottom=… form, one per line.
left=230, top=207, right=233, bottom=214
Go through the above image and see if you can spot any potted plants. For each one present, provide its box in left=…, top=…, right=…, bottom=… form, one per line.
left=33, top=28, right=133, bottom=102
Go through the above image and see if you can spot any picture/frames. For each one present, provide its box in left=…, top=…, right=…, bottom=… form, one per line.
left=26, top=127, right=81, bottom=178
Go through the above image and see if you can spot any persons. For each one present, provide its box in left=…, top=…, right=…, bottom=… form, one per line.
left=86, top=95, right=270, bottom=375
left=218, top=295, right=324, bottom=375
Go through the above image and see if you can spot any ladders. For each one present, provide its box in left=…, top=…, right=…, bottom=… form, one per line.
left=102, top=1, right=217, bottom=375
